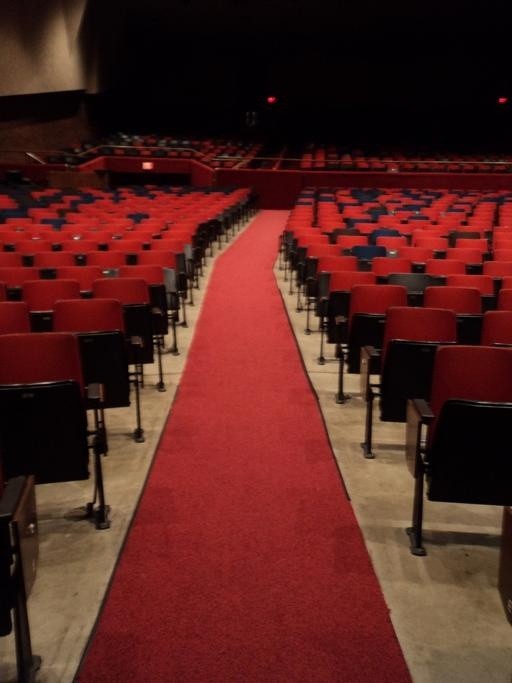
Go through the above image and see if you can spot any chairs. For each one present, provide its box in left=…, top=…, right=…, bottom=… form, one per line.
left=279, top=186, right=511, bottom=622
left=70, top=133, right=508, bottom=175
left=2, top=186, right=253, bottom=683
